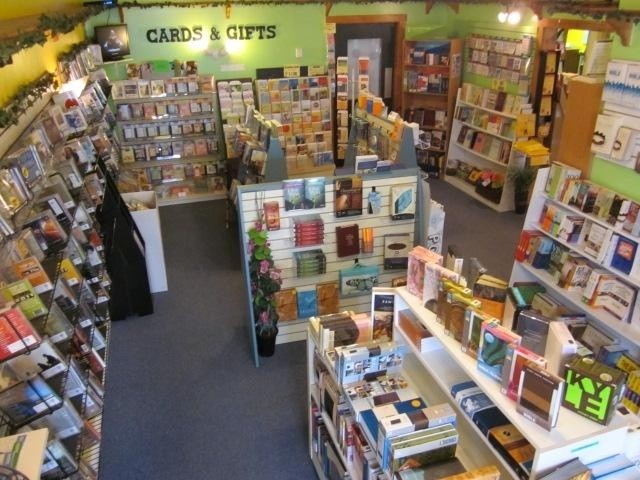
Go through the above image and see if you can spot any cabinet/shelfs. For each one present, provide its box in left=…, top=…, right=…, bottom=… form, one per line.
left=402, top=36, right=533, bottom=214
left=110, top=57, right=371, bottom=208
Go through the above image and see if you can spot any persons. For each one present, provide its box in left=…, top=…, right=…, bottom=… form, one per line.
left=310, top=243, right=534, bottom=479
left=103, top=30, right=123, bottom=51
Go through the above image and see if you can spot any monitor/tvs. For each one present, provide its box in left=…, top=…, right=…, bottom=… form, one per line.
left=92, top=24, right=130, bottom=63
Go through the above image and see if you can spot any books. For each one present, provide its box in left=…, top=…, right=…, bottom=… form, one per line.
left=264, top=176, right=417, bottom=324
left=508, top=160, right=640, bottom=434
left=533, top=451, right=640, bottom=480
left=111, top=55, right=404, bottom=204
left=402, top=34, right=557, bottom=186
left=589, top=59, right=639, bottom=171
left=0, top=43, right=122, bottom=479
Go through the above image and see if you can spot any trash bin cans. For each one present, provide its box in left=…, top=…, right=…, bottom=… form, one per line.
left=119, top=191, right=168, bottom=294
